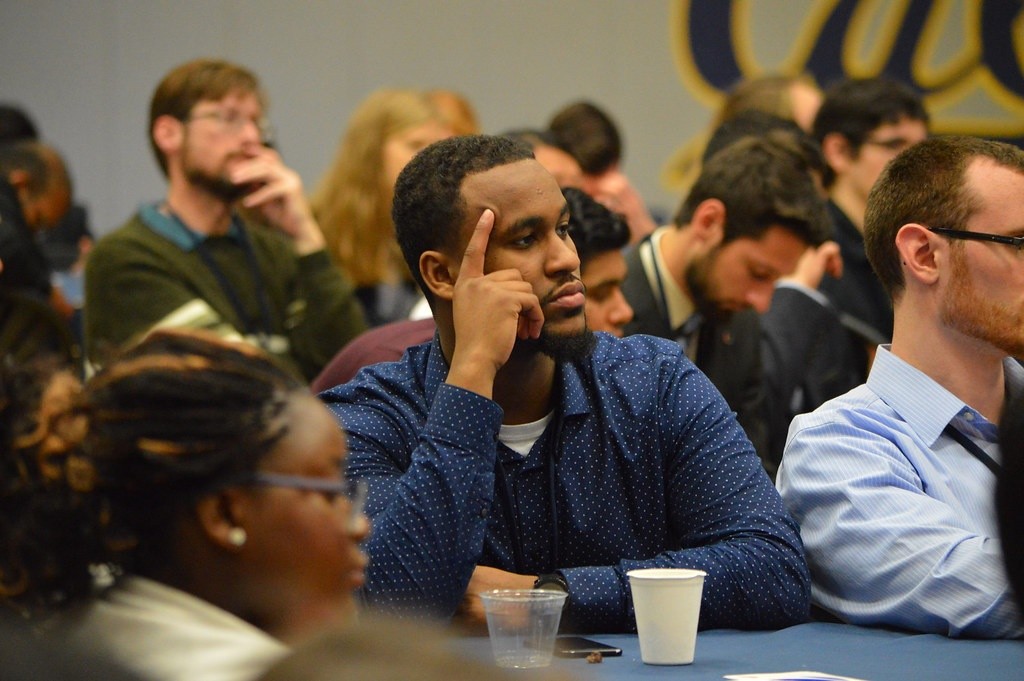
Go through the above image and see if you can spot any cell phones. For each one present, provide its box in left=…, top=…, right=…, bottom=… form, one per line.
left=524, top=636, right=622, bottom=659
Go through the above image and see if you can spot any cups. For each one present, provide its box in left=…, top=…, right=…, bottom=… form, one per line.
left=626, top=567, right=707, bottom=665
left=478, top=588, right=569, bottom=670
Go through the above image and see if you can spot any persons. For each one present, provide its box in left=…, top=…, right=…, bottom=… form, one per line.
left=0, top=61, right=1024, bottom=680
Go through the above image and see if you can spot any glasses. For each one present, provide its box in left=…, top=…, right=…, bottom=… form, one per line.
left=191, top=108, right=269, bottom=134
left=216, top=472, right=369, bottom=515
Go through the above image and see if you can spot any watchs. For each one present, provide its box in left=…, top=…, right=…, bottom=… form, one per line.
left=529, top=573, right=571, bottom=636
